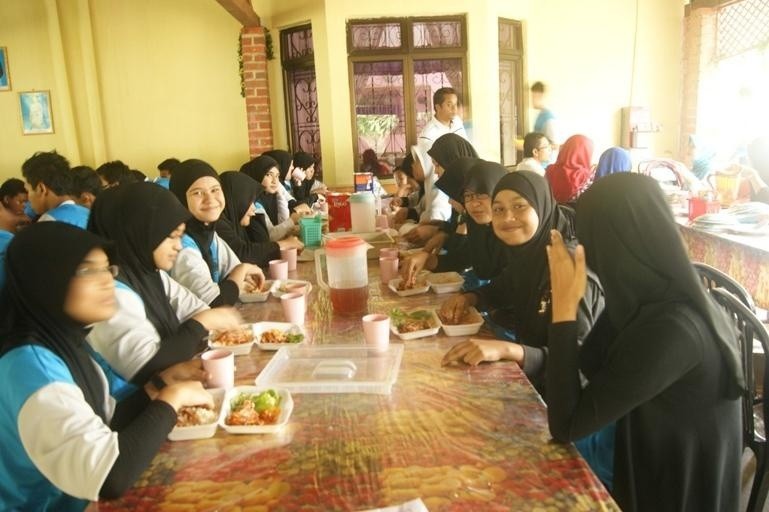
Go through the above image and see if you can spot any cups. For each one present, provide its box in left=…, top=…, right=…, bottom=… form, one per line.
left=379, top=256, right=399, bottom=286
left=284, top=282, right=308, bottom=313
left=280, top=246, right=297, bottom=272
left=382, top=207, right=388, bottom=214
left=380, top=247, right=399, bottom=255
left=281, top=292, right=305, bottom=327
left=268, top=259, right=289, bottom=282
left=380, top=215, right=388, bottom=229
left=361, top=313, right=391, bottom=353
left=200, top=349, right=235, bottom=390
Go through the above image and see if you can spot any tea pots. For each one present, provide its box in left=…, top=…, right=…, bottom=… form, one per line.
left=314, top=237, right=376, bottom=316
left=345, top=192, right=382, bottom=235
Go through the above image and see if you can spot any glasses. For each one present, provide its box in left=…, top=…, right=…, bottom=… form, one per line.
left=74, top=264, right=120, bottom=282
left=461, top=190, right=490, bottom=202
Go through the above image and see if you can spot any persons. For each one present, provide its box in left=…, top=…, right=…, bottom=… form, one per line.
left=357, top=83, right=769, bottom=511
left=29, top=96, right=43, bottom=128
left=1, top=153, right=328, bottom=512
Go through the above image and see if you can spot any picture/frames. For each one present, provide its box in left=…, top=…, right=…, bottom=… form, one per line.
left=0, top=46, right=15, bottom=94
left=15, top=89, right=54, bottom=137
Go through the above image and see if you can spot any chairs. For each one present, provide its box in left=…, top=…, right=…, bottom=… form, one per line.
left=636, top=158, right=684, bottom=187
left=687, top=259, right=758, bottom=444
left=707, top=285, right=769, bottom=512
